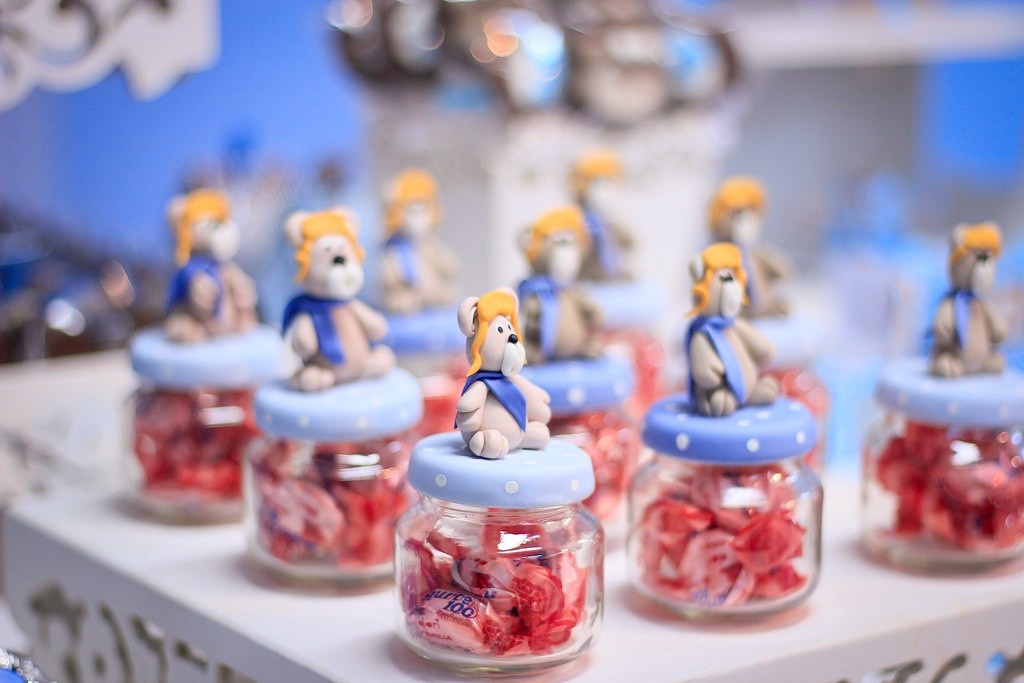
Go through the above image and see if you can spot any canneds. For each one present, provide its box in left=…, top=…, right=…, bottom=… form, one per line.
left=127, top=300, right=1024, bottom=672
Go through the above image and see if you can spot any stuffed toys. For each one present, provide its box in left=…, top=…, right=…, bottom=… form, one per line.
left=141, top=152, right=1008, bottom=462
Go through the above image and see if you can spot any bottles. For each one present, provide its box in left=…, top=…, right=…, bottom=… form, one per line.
left=393, top=429, right=606, bottom=675
left=635, top=304, right=820, bottom=447
left=537, top=278, right=662, bottom=408
left=858, top=357, right=1024, bottom=577
left=238, top=365, right=424, bottom=582
left=354, top=299, right=483, bottom=437
left=115, top=320, right=284, bottom=519
left=621, top=390, right=823, bottom=616
left=506, top=338, right=644, bottom=505
